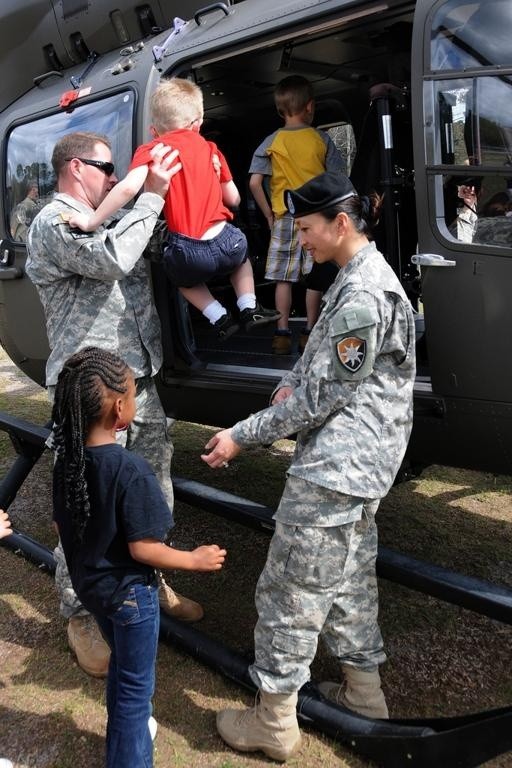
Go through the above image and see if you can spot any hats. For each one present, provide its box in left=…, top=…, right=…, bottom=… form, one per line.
left=284, top=168, right=357, bottom=219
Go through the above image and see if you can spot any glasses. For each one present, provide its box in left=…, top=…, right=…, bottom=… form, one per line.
left=63, top=153, right=115, bottom=176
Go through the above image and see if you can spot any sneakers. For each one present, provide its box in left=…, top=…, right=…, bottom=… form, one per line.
left=273, top=329, right=293, bottom=354
left=298, top=333, right=311, bottom=346
left=67, top=613, right=112, bottom=677
left=156, top=569, right=205, bottom=623
left=239, top=300, right=282, bottom=329
left=212, top=308, right=240, bottom=340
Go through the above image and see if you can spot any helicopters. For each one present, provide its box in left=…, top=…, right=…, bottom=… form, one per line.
left=1, top=0, right=512, bottom=768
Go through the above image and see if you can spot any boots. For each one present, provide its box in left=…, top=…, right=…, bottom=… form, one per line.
left=216, top=691, right=302, bottom=760
left=315, top=666, right=389, bottom=720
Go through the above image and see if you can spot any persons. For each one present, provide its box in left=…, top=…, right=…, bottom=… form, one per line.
left=0, top=509, right=14, bottom=540
left=69, top=76, right=282, bottom=343
left=49, top=345, right=226, bottom=768
left=198, top=168, right=417, bottom=762
left=24, top=133, right=205, bottom=679
left=248, top=75, right=344, bottom=356
left=9, top=178, right=42, bottom=243
